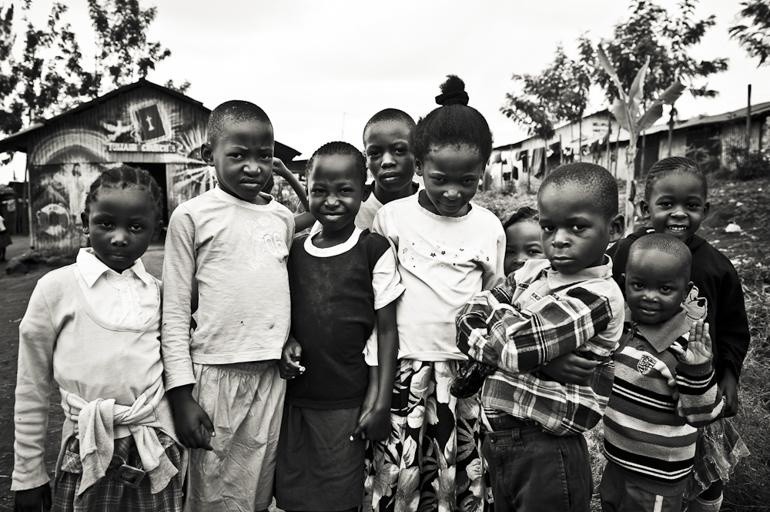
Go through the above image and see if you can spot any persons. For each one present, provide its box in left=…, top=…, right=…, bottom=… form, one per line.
left=502, top=208, right=544, bottom=278
left=360, top=75, right=507, bottom=511
left=593, top=232, right=727, bottom=511
left=358, top=110, right=421, bottom=231
left=274, top=142, right=406, bottom=510
left=607, top=159, right=748, bottom=510
left=162, top=101, right=294, bottom=511
left=455, top=163, right=624, bottom=510
left=69, top=163, right=84, bottom=226
left=10, top=170, right=181, bottom=511
